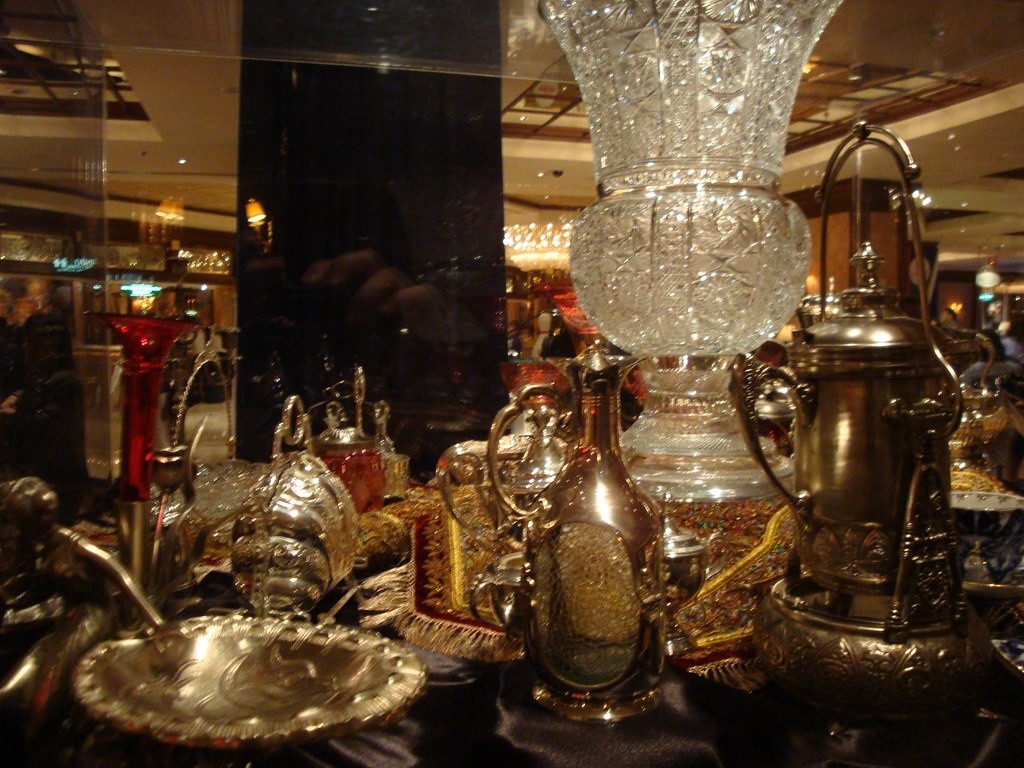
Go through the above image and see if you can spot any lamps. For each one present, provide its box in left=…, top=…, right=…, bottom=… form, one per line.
left=246, top=199, right=273, bottom=260
left=154, top=198, right=184, bottom=220
left=503, top=222, right=571, bottom=272
left=974, top=266, right=1002, bottom=287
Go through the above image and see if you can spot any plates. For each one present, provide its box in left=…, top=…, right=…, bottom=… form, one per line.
left=78, top=611, right=423, bottom=746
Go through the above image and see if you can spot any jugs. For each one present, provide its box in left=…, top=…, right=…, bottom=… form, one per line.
left=482, top=351, right=672, bottom=718
left=736, top=116, right=995, bottom=698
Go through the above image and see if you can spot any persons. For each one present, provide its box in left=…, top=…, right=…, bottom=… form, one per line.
left=995, top=321, right=1024, bottom=357
left=957, top=327, right=1024, bottom=388
left=0, top=273, right=90, bottom=525
